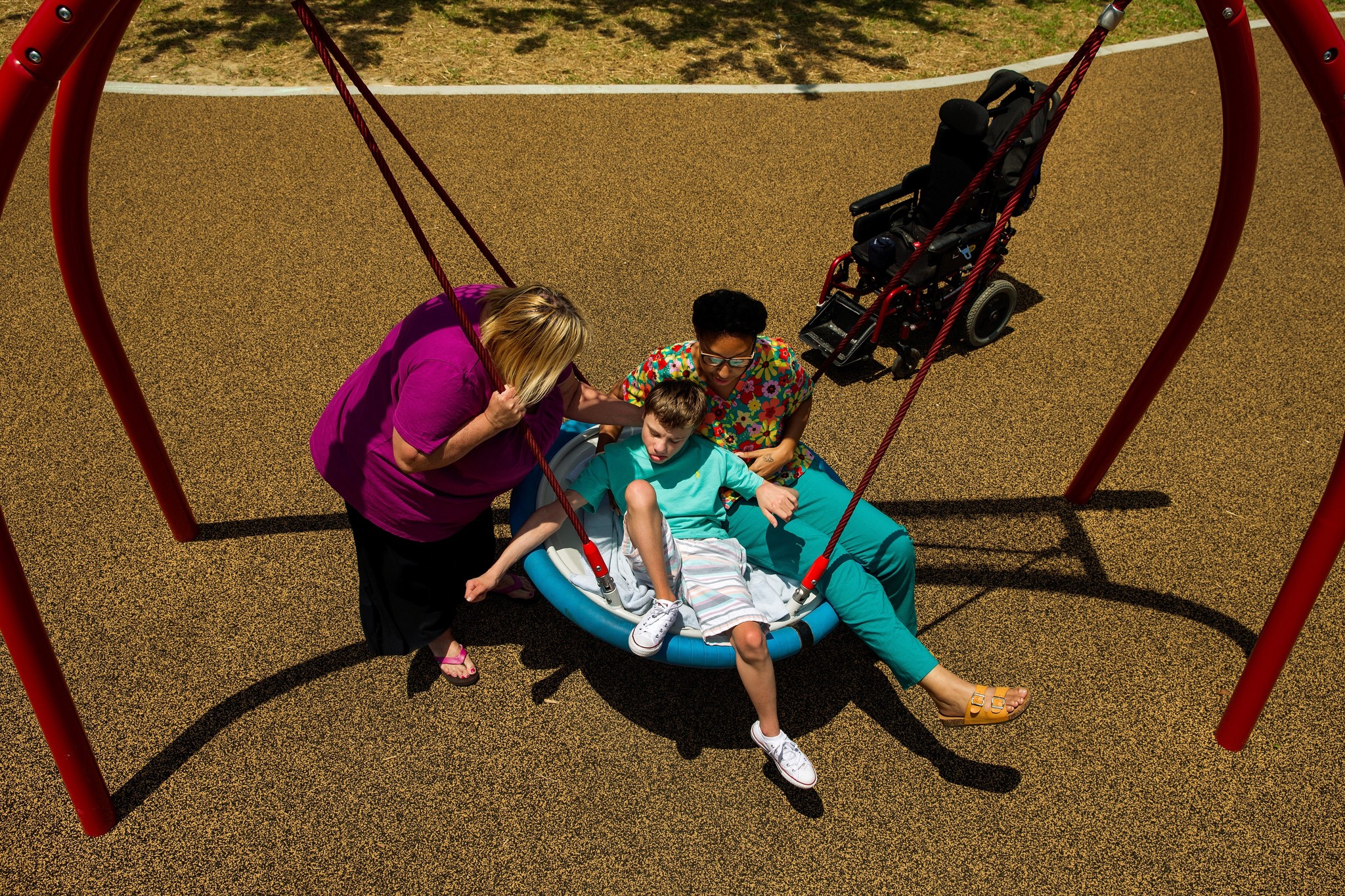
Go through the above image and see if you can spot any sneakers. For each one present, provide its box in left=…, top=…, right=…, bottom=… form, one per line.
left=750, top=721, right=817, bottom=789
left=629, top=596, right=683, bottom=657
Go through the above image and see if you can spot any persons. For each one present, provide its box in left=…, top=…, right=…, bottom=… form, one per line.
left=311, top=283, right=634, bottom=688
left=462, top=379, right=821, bottom=789
left=586, top=289, right=1031, bottom=727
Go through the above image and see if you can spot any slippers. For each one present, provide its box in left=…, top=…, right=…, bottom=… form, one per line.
left=491, top=573, right=536, bottom=603
left=433, top=643, right=479, bottom=688
left=938, top=688, right=1031, bottom=726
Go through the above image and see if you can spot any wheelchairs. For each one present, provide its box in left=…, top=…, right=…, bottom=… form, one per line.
left=799, top=70, right=1062, bottom=366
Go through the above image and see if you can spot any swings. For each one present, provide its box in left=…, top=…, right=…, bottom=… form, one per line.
left=290, top=0, right=1135, bottom=670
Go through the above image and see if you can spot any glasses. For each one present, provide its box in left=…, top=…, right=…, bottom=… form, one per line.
left=698, top=338, right=755, bottom=368
